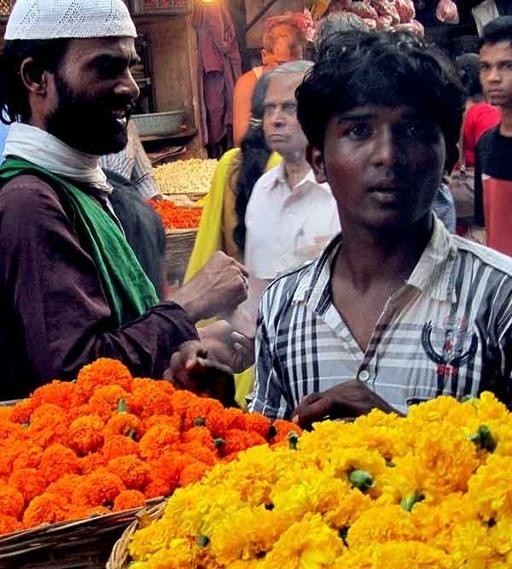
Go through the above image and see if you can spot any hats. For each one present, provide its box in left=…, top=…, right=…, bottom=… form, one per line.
left=4, top=1, right=139, bottom=41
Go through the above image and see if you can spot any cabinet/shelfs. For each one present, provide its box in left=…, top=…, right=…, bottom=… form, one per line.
left=130, top=8, right=196, bottom=142
left=133, top=31, right=158, bottom=114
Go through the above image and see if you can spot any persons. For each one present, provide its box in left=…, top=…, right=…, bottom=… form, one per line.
left=160, top=26, right=512, bottom=432
left=0, top=0, right=253, bottom=406
left=180, top=1, right=512, bottom=403
left=97, top=118, right=166, bottom=207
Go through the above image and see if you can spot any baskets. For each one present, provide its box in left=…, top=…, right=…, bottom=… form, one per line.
left=1, top=395, right=174, bottom=569
left=160, top=192, right=207, bottom=275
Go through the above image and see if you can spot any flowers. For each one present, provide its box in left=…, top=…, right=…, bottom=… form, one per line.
left=126, top=390, right=512, bottom=569
left=0, top=357, right=302, bottom=534
